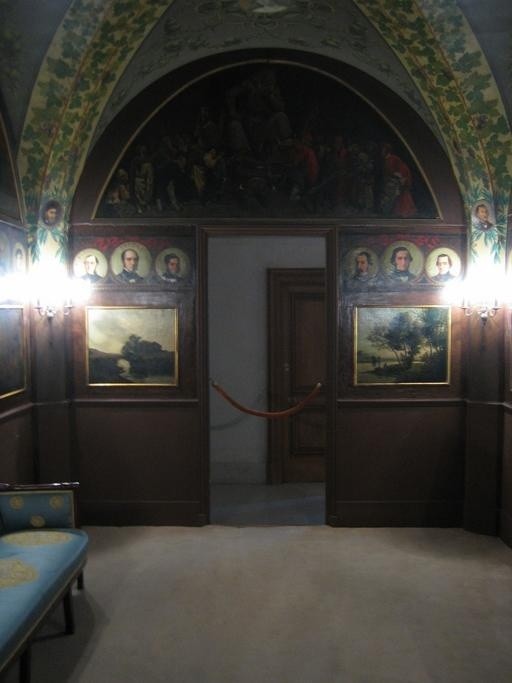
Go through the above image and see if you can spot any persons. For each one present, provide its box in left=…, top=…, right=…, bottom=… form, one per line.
left=43, top=201, right=60, bottom=229
left=161, top=252, right=183, bottom=280
left=387, top=246, right=417, bottom=280
left=347, top=251, right=374, bottom=280
left=15, top=248, right=26, bottom=276
left=428, top=253, right=457, bottom=282
left=117, top=248, right=144, bottom=281
left=82, top=253, right=104, bottom=282
left=475, top=201, right=492, bottom=231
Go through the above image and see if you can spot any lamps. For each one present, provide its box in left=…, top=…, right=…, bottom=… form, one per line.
left=31, top=293, right=76, bottom=321
left=458, top=296, right=504, bottom=326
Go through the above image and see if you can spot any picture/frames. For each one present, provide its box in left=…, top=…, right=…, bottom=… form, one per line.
left=351, top=303, right=453, bottom=386
left=68, top=223, right=199, bottom=295
left=336, top=222, right=467, bottom=296
left=84, top=303, right=179, bottom=387
left=0, top=303, right=33, bottom=400
left=1, top=212, right=32, bottom=303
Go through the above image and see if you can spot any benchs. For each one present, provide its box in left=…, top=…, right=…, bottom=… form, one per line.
left=1, top=479, right=89, bottom=682
